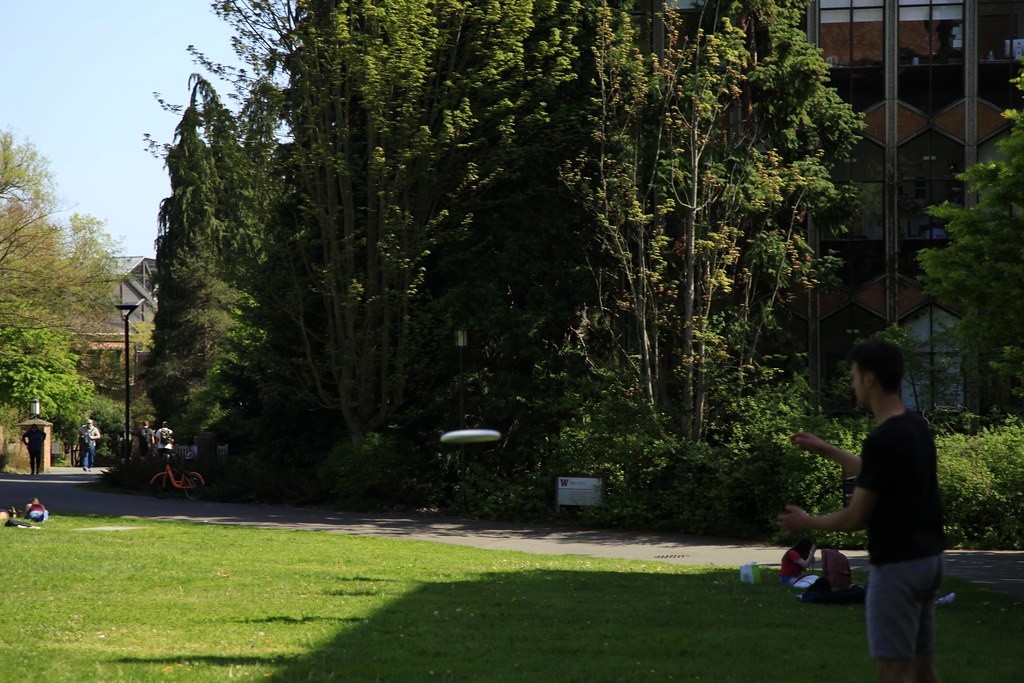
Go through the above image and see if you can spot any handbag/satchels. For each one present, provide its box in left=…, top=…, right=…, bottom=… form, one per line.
left=838, top=584, right=865, bottom=602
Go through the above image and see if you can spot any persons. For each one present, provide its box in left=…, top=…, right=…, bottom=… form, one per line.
left=117, top=422, right=136, bottom=465
left=136, top=420, right=155, bottom=457
left=78, top=419, right=100, bottom=472
left=191, top=435, right=199, bottom=459
left=155, top=421, right=175, bottom=457
left=25, top=497, right=48, bottom=522
left=796, top=576, right=955, bottom=609
left=780, top=538, right=819, bottom=588
left=775, top=340, right=946, bottom=683
left=22, top=424, right=46, bottom=475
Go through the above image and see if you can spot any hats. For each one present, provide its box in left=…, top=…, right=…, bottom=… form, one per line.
left=31, top=424, right=38, bottom=428
left=86, top=419, right=93, bottom=424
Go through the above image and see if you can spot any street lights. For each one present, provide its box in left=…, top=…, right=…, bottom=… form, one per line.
left=452, top=324, right=474, bottom=521
left=113, top=302, right=142, bottom=463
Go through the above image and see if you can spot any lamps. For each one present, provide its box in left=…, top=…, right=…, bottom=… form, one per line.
left=30, top=399, right=40, bottom=418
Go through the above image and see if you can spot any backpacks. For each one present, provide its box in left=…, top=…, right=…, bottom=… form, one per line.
left=801, top=578, right=829, bottom=605
left=139, top=428, right=149, bottom=446
left=160, top=427, right=171, bottom=445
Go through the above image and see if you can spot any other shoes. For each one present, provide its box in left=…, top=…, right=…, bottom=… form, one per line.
left=83, top=467, right=87, bottom=471
left=89, top=469, right=92, bottom=471
left=939, top=593, right=955, bottom=607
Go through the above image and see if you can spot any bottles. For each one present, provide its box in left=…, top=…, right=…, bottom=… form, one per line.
left=935, top=592, right=955, bottom=605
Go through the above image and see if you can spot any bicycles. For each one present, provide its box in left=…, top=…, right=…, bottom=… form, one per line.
left=147, top=449, right=206, bottom=500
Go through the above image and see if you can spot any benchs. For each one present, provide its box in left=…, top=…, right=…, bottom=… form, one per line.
left=175, top=442, right=229, bottom=457
